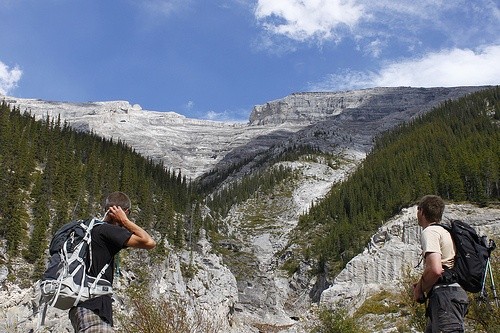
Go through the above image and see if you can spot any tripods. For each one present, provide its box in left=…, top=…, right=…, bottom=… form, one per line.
left=478, top=239, right=499, bottom=308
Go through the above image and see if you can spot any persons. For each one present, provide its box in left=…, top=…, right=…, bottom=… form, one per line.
left=67, top=190, right=158, bottom=333
left=413, top=194, right=472, bottom=333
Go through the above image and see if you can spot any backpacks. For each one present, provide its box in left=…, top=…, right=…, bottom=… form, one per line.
left=39, top=218, right=113, bottom=325
left=430, top=219, right=490, bottom=293
